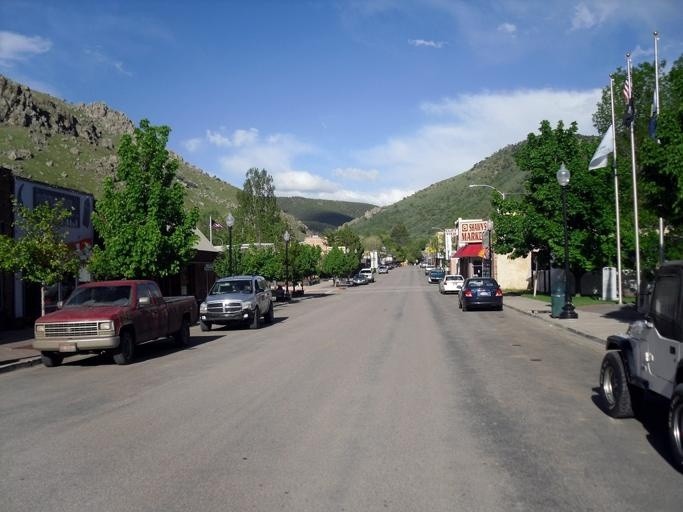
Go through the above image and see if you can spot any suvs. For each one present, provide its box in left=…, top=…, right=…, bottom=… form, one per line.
left=199, top=275, right=274, bottom=331
left=359, top=268, right=374, bottom=282
left=599, top=259, right=683, bottom=461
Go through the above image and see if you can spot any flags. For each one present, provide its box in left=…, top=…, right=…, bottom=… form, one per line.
left=588, top=125, right=614, bottom=171
left=649, top=88, right=662, bottom=145
left=623, top=59, right=634, bottom=127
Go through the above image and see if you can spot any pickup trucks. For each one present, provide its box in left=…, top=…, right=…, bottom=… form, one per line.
left=32, top=280, right=199, bottom=366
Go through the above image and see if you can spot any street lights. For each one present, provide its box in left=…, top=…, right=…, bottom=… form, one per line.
left=557, top=160, right=579, bottom=319
left=470, top=184, right=504, bottom=199
left=284, top=230, right=291, bottom=302
left=226, top=213, right=235, bottom=277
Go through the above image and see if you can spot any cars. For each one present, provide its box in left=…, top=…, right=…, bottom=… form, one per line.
left=457, top=277, right=502, bottom=311
left=379, top=263, right=393, bottom=274
left=421, top=262, right=445, bottom=284
left=352, top=273, right=369, bottom=285
left=439, top=275, right=465, bottom=294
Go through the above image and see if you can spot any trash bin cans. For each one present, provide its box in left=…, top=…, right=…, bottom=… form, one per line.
left=551, top=294, right=565, bottom=318
